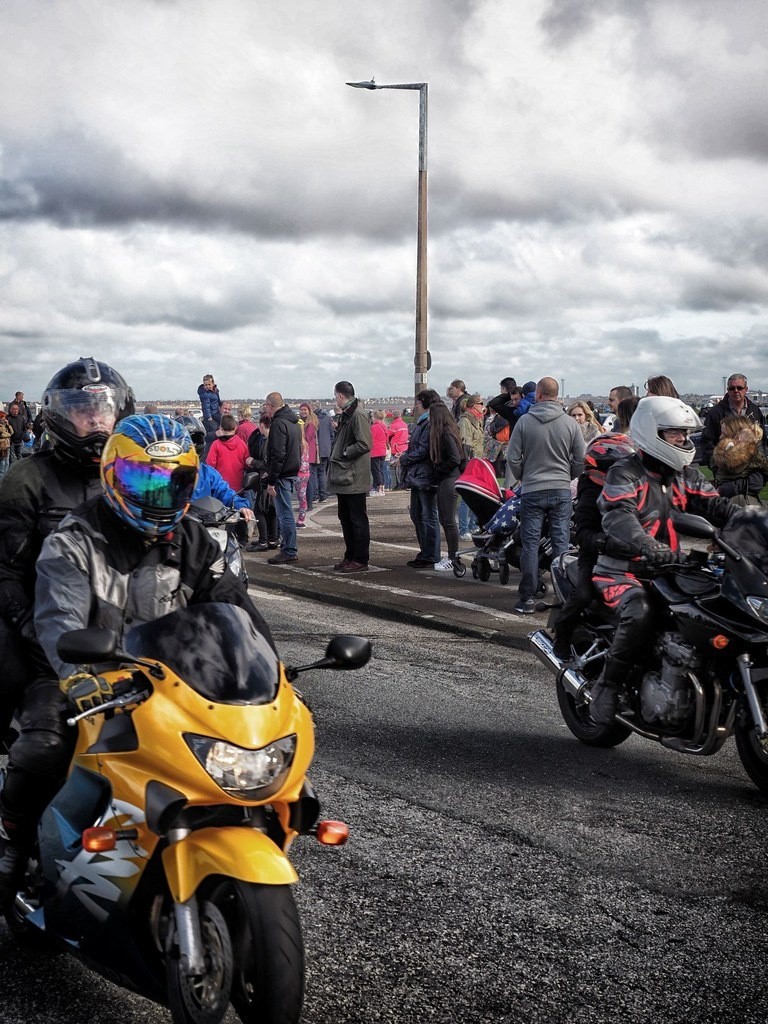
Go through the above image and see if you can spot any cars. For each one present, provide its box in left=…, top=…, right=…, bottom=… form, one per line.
left=189, top=409, right=203, bottom=420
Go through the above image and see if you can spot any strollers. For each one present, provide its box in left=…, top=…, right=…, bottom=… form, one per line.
left=455, top=458, right=549, bottom=584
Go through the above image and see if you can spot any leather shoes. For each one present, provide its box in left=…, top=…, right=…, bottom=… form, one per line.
left=267, top=550, right=298, bottom=564
left=334, top=557, right=369, bottom=573
left=246, top=539, right=281, bottom=552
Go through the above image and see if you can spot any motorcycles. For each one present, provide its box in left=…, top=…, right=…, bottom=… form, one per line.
left=525, top=505, right=768, bottom=799
left=0, top=603, right=371, bottom=1024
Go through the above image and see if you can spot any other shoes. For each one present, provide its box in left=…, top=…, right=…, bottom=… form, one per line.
left=295, top=498, right=326, bottom=527
left=489, top=557, right=501, bottom=571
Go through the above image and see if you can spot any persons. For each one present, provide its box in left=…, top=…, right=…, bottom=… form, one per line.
left=506, top=377, right=587, bottom=614
left=0, top=358, right=143, bottom=901
left=0, top=374, right=768, bottom=573
left=589, top=396, right=745, bottom=719
left=33, top=413, right=294, bottom=723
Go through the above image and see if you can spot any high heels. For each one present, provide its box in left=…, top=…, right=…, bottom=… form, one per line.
left=551, top=616, right=572, bottom=660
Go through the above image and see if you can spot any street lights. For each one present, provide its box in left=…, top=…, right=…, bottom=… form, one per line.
left=344, top=82, right=429, bottom=405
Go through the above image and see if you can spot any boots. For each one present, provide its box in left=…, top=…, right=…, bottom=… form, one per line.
left=0, top=790, right=33, bottom=882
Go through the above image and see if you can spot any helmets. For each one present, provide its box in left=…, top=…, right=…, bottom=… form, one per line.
left=99, top=414, right=199, bottom=539
left=584, top=432, right=639, bottom=471
left=630, top=396, right=706, bottom=472
left=175, top=416, right=207, bottom=457
left=41, top=357, right=137, bottom=474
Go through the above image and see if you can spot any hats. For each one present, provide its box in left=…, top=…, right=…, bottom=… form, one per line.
left=300, top=403, right=310, bottom=411
left=0, top=411, right=8, bottom=418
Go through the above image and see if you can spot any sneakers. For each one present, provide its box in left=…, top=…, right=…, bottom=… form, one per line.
left=590, top=673, right=618, bottom=725
left=407, top=559, right=434, bottom=569
left=514, top=597, right=535, bottom=614
left=434, top=557, right=455, bottom=571
left=459, top=529, right=480, bottom=542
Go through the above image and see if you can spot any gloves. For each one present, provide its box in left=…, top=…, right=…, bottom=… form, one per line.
left=58, top=663, right=117, bottom=721
left=631, top=534, right=676, bottom=564
left=591, top=532, right=633, bottom=561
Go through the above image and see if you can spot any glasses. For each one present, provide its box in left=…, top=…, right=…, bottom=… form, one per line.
left=263, top=404, right=274, bottom=407
left=728, top=385, right=746, bottom=391
left=477, top=401, right=483, bottom=405
left=663, top=429, right=688, bottom=437
